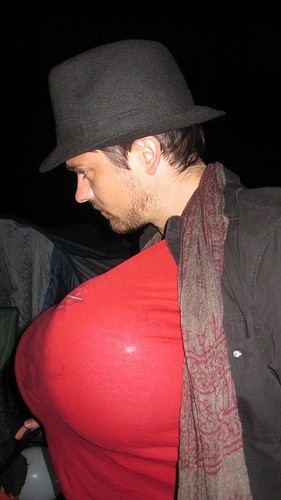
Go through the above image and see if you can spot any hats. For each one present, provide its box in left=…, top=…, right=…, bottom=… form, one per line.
left=39, top=39, right=226, bottom=174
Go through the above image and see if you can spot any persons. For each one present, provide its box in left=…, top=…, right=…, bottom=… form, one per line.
left=14, top=37, right=281, bottom=500
